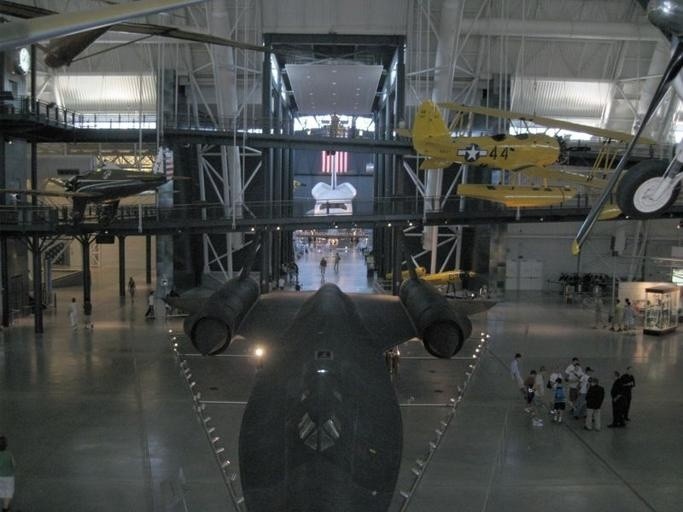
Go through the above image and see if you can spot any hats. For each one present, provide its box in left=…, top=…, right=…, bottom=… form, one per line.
left=586, top=367, right=593, bottom=372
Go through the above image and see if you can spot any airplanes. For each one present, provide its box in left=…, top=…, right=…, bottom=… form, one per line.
left=404, top=98, right=683, bottom=258
left=157, top=270, right=500, bottom=511
left=40, top=162, right=172, bottom=235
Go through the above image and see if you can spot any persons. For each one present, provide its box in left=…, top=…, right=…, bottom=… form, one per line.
left=145, top=290, right=156, bottom=317
left=81, top=294, right=94, bottom=330
left=508, top=353, right=637, bottom=434
left=0, top=436, right=18, bottom=512
left=163, top=294, right=173, bottom=319
left=260, top=229, right=380, bottom=292
left=127, top=276, right=136, bottom=301
left=588, top=293, right=665, bottom=337
left=65, top=297, right=79, bottom=333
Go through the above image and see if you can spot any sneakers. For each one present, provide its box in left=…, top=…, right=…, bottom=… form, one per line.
left=607, top=417, right=629, bottom=427
left=550, top=409, right=563, bottom=423
left=524, top=407, right=535, bottom=413
left=533, top=418, right=544, bottom=427
